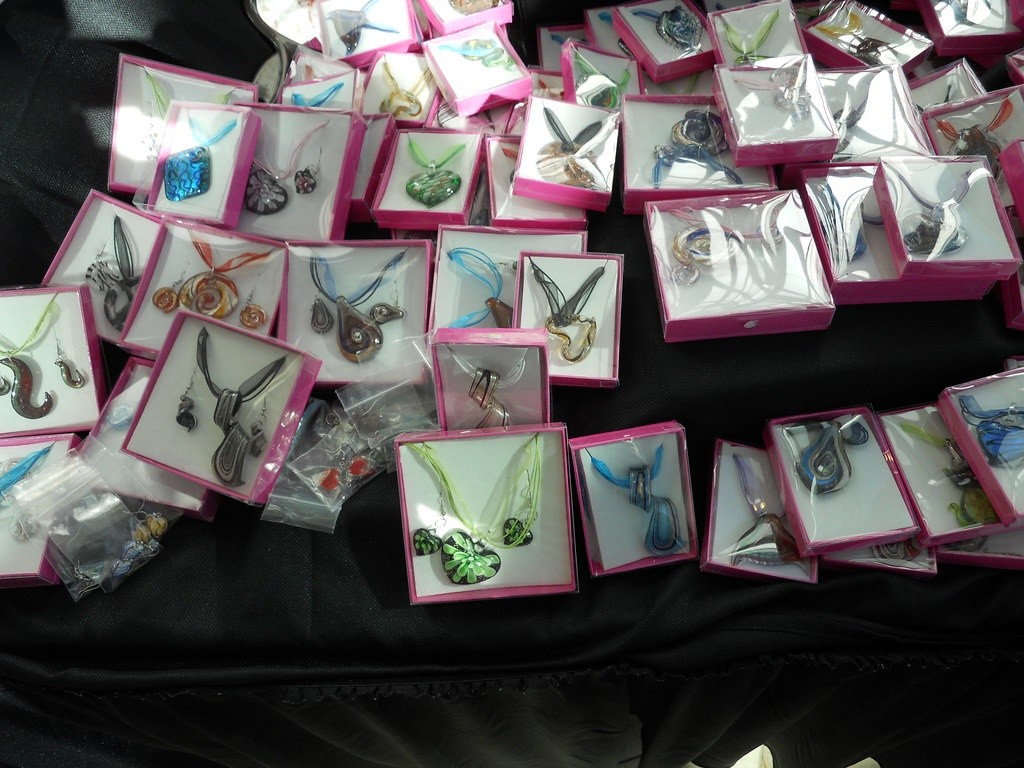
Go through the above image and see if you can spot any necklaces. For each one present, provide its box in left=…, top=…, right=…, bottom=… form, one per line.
left=1, top=441, right=54, bottom=545
left=959, top=385, right=1024, bottom=469
left=0, top=293, right=85, bottom=418
left=44, top=0, right=1024, bottom=430
left=582, top=440, right=688, bottom=557
left=408, top=429, right=545, bottom=585
left=729, top=452, right=801, bottom=569
left=796, top=417, right=870, bottom=494
left=177, top=327, right=287, bottom=490
left=899, top=413, right=1000, bottom=528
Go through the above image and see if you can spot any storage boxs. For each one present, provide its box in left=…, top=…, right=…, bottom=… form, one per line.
left=0, top=0, right=1023, bottom=602
